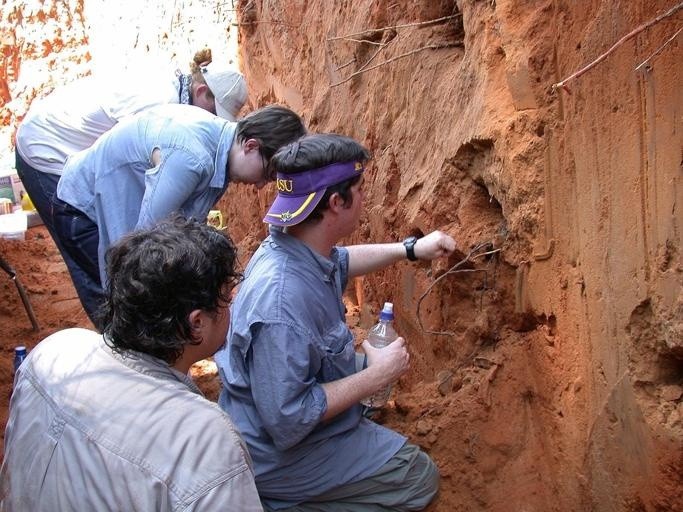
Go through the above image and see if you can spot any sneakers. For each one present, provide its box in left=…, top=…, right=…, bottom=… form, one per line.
left=189, top=359, right=217, bottom=383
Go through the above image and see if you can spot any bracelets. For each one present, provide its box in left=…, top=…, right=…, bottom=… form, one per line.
left=402, top=234, right=424, bottom=262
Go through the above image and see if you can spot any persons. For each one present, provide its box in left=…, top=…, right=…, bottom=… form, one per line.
left=198, top=128, right=460, bottom=510
left=18, top=190, right=35, bottom=212
left=1, top=214, right=276, bottom=511
left=12, top=56, right=246, bottom=338
left=40, top=97, right=308, bottom=331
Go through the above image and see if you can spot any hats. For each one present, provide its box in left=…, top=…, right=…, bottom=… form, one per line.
left=260, top=159, right=363, bottom=228
left=199, top=62, right=248, bottom=122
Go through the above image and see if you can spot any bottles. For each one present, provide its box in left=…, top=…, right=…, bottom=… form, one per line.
left=13, top=346, right=27, bottom=370
left=358, top=301, right=399, bottom=409
